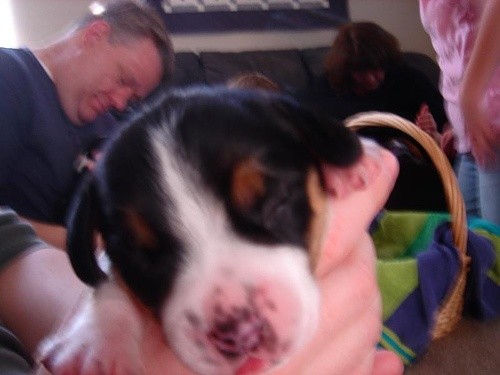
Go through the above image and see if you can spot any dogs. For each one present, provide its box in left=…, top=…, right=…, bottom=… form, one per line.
left=35, top=85, right=390, bottom=375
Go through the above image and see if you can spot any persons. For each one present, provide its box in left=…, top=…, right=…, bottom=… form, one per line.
left=414, top=1, right=500, bottom=226
left=0, top=147, right=405, bottom=375
left=232, top=71, right=275, bottom=95
left=0, top=1, right=180, bottom=248
left=325, top=15, right=458, bottom=213
